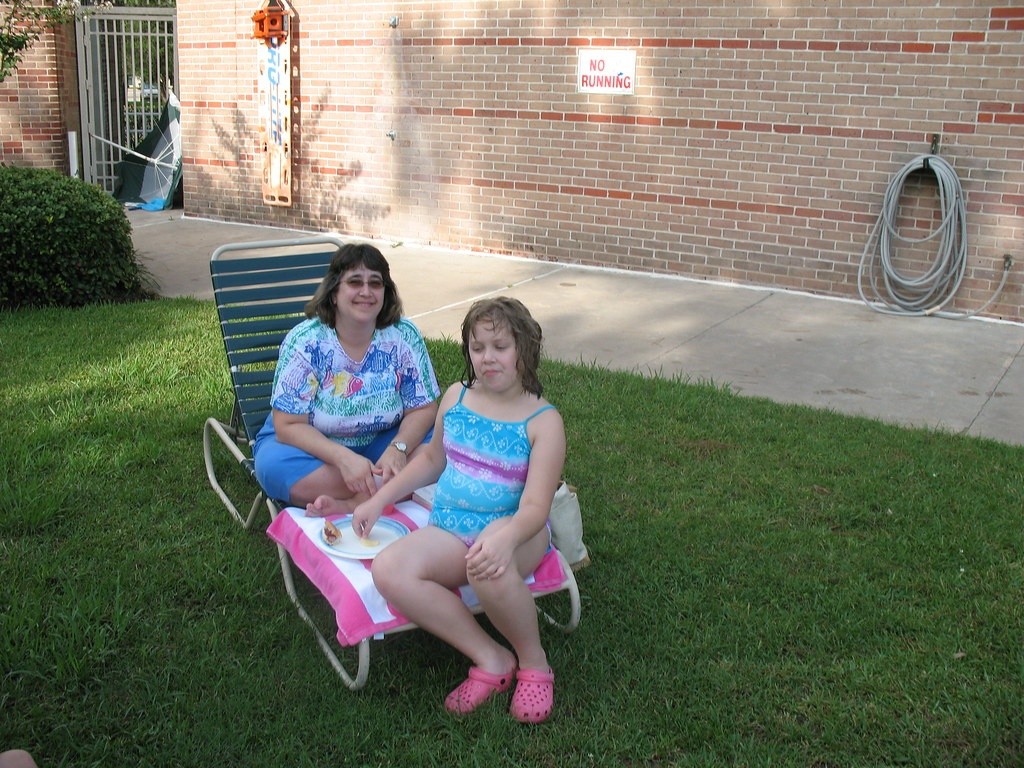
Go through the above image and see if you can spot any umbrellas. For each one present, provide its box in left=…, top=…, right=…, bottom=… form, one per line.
left=90, top=91, right=183, bottom=211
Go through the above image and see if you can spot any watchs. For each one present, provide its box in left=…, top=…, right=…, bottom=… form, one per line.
left=388, top=440, right=408, bottom=456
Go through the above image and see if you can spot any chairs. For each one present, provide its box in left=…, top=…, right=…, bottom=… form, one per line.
left=202, top=236, right=582, bottom=690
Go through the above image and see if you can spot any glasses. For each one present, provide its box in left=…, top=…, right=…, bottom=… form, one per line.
left=337, top=276, right=389, bottom=290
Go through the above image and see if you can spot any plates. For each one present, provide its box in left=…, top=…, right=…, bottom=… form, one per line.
left=318, top=517, right=411, bottom=560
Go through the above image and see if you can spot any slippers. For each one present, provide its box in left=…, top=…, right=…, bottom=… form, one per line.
left=445, top=665, right=517, bottom=716
left=511, top=667, right=555, bottom=722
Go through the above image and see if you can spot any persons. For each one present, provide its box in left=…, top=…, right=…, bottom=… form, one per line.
left=253, top=243, right=448, bottom=518
left=351, top=297, right=566, bottom=723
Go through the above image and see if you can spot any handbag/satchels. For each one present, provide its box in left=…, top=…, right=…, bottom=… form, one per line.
left=548, top=480, right=591, bottom=572
